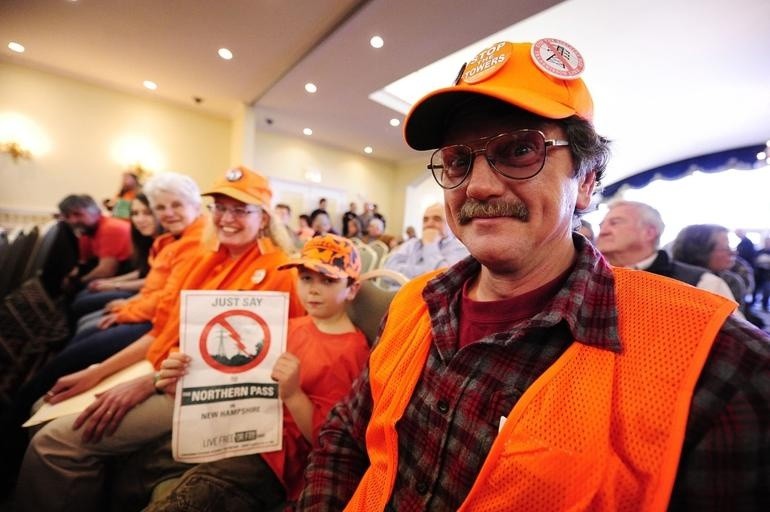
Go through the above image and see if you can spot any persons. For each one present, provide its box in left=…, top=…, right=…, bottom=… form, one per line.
left=378, top=202, right=472, bottom=293
left=672, top=224, right=746, bottom=315
left=104, top=169, right=143, bottom=211
left=595, top=201, right=757, bottom=331
left=41, top=172, right=219, bottom=390
left=15, top=166, right=306, bottom=512
left=734, top=228, right=756, bottom=267
left=57, top=194, right=136, bottom=291
left=75, top=190, right=164, bottom=341
left=300, top=37, right=770, bottom=512
left=139, top=236, right=371, bottom=512
left=274, top=197, right=419, bottom=252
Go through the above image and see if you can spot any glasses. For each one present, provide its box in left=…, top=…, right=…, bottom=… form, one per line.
left=214, top=203, right=260, bottom=217
left=427, top=128, right=569, bottom=189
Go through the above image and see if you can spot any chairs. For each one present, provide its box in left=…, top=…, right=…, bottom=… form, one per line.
left=376, top=252, right=388, bottom=289
left=345, top=269, right=411, bottom=348
left=22, top=221, right=56, bottom=313
left=357, top=244, right=377, bottom=274
left=368, top=240, right=390, bottom=270
left=5, top=225, right=50, bottom=344
left=7, top=224, right=50, bottom=327
left=349, top=237, right=362, bottom=246
left=0, top=229, right=26, bottom=373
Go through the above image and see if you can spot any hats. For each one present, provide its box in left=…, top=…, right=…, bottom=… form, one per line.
left=277, top=233, right=361, bottom=279
left=402, top=38, right=594, bottom=151
left=200, top=165, right=273, bottom=211
